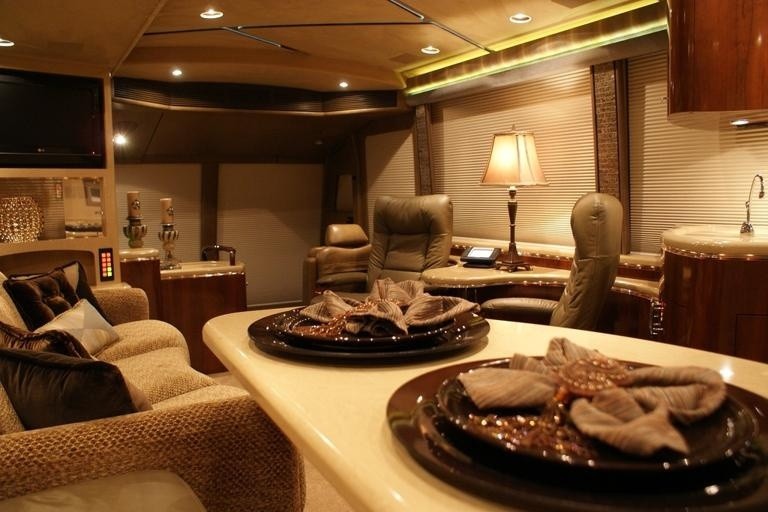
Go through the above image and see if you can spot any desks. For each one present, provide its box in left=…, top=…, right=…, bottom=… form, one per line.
left=198, top=304, right=767, bottom=511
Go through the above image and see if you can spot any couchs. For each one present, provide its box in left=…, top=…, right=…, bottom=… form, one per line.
left=0, top=269, right=307, bottom=512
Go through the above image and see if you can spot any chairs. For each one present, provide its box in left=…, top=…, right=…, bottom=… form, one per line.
left=478, top=191, right=631, bottom=333
left=302, top=222, right=371, bottom=298
left=364, top=194, right=455, bottom=299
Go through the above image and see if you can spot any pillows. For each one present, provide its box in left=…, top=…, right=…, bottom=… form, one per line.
left=31, top=294, right=122, bottom=358
left=125, top=377, right=151, bottom=411
left=0, top=348, right=136, bottom=434
left=0, top=261, right=121, bottom=335
left=0, top=319, right=98, bottom=359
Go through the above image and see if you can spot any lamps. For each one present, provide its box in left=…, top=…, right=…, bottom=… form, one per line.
left=478, top=122, right=551, bottom=273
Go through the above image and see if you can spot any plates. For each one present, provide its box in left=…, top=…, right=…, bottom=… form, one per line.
left=437, top=360, right=757, bottom=472
left=248, top=311, right=490, bottom=359
left=387, top=358, right=768, bottom=512
left=276, top=313, right=458, bottom=344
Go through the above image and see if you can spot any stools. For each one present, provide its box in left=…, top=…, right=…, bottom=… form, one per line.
left=0, top=467, right=211, bottom=512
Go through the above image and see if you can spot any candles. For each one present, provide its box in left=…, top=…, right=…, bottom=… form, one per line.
left=159, top=196, right=175, bottom=224
left=127, top=189, right=143, bottom=218
